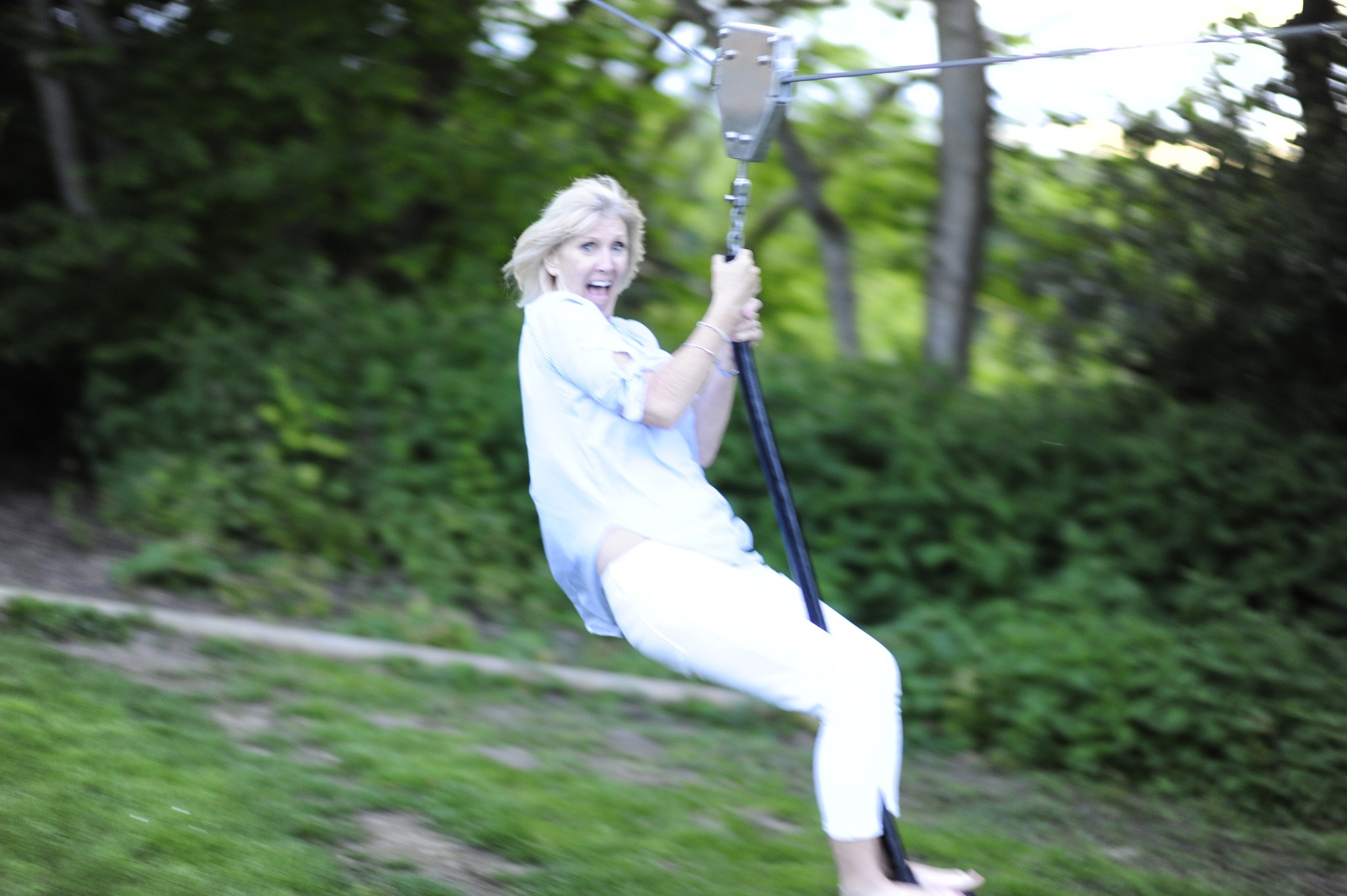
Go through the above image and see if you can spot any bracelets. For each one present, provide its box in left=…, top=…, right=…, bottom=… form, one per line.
left=679, top=344, right=717, bottom=359
left=713, top=361, right=739, bottom=377
left=696, top=321, right=731, bottom=344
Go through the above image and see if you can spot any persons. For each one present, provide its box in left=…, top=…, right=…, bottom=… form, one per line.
left=500, top=176, right=983, bottom=896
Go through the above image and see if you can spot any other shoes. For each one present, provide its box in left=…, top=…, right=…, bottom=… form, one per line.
left=838, top=859, right=985, bottom=896
